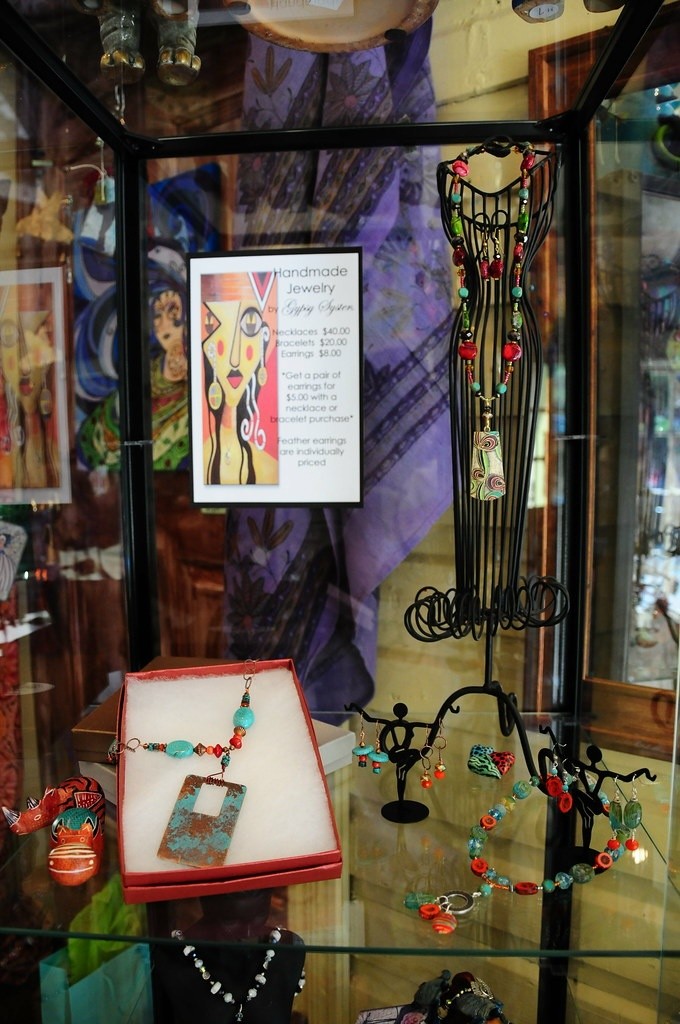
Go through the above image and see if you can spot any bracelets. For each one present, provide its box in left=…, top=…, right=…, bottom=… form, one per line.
left=403, top=770, right=626, bottom=936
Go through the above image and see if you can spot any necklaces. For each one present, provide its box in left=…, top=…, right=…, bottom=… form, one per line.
left=170, top=923, right=305, bottom=1022
left=449, top=132, right=536, bottom=504
left=106, top=657, right=258, bottom=867
